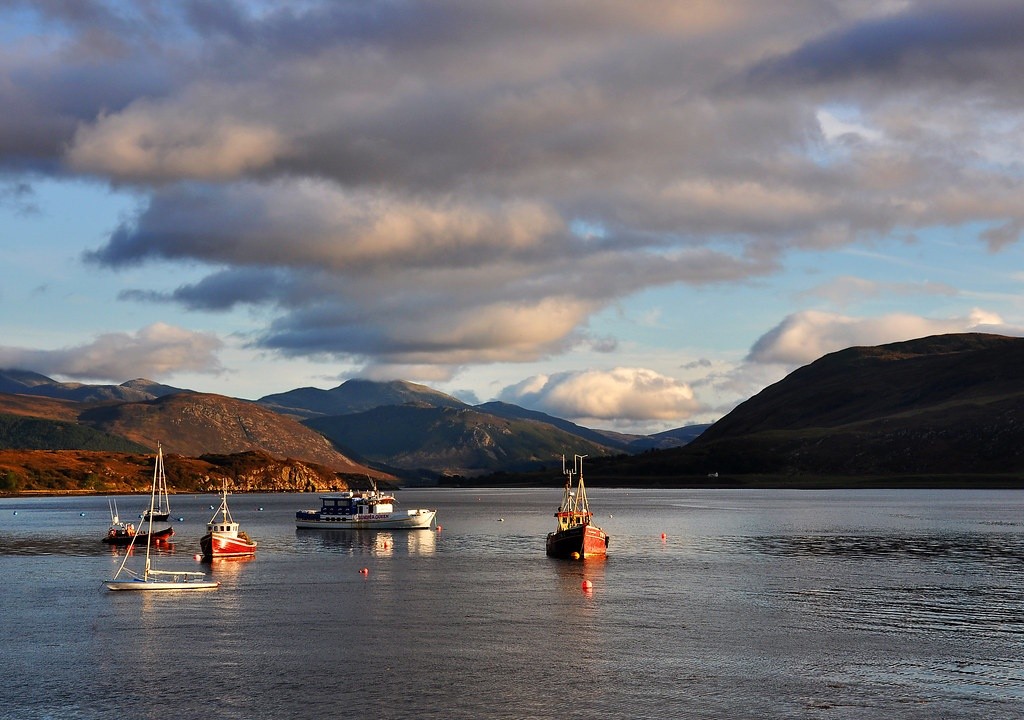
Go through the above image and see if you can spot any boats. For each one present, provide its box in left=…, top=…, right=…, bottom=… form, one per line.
left=545, top=451, right=610, bottom=560
left=295, top=474, right=437, bottom=531
left=200, top=478, right=257, bottom=557
left=101, top=499, right=174, bottom=544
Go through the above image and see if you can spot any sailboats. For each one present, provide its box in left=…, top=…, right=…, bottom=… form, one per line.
left=99, top=455, right=219, bottom=590
left=138, top=439, right=171, bottom=523
